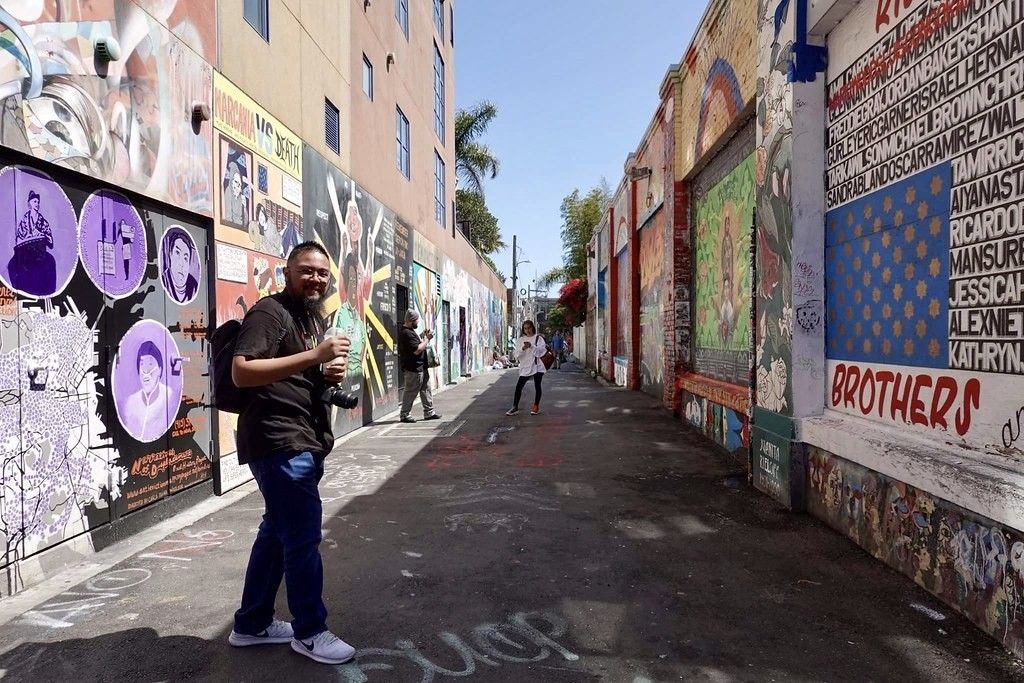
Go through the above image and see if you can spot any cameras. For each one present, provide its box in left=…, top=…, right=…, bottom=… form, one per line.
left=313, top=381, right=359, bottom=409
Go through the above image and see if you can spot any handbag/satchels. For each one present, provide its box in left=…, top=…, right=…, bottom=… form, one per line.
left=536, top=335, right=556, bottom=370
left=426, top=343, right=440, bottom=367
left=560, top=353, right=567, bottom=364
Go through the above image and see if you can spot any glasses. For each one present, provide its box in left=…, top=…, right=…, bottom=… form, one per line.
left=286, top=267, right=331, bottom=279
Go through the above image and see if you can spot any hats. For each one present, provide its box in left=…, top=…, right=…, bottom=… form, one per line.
left=404, top=308, right=419, bottom=322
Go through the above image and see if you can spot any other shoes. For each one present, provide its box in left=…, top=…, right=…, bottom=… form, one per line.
left=558, top=367, right=561, bottom=369
left=425, top=414, right=442, bottom=419
left=510, top=364, right=512, bottom=368
left=552, top=367, right=557, bottom=369
left=514, top=365, right=518, bottom=367
left=402, top=417, right=417, bottom=422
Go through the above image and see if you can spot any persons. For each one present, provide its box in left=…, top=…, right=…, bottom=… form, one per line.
left=551, top=329, right=563, bottom=369
left=228, top=242, right=355, bottom=666
left=493, top=345, right=514, bottom=367
left=506, top=320, right=547, bottom=416
left=565, top=332, right=572, bottom=354
left=397, top=307, right=442, bottom=423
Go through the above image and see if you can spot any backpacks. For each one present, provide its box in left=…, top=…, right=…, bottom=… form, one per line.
left=206, top=292, right=311, bottom=414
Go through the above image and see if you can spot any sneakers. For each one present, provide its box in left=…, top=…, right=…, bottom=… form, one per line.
left=530, top=404, right=540, bottom=414
left=505, top=404, right=519, bottom=415
left=229, top=618, right=294, bottom=646
left=291, top=630, right=355, bottom=664
